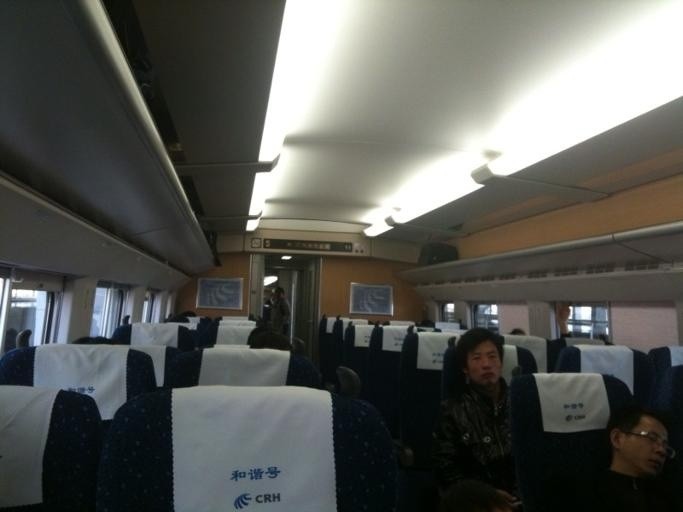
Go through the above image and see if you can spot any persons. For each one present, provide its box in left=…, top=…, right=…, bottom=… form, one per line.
left=508, top=328, right=526, bottom=335
left=580, top=403, right=683, bottom=512
left=432, top=326, right=528, bottom=511
left=263, top=286, right=291, bottom=339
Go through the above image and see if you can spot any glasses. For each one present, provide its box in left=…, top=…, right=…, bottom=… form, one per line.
left=621, top=427, right=677, bottom=459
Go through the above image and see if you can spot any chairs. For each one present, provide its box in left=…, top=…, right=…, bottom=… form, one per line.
left=0, top=314, right=680, bottom=512
